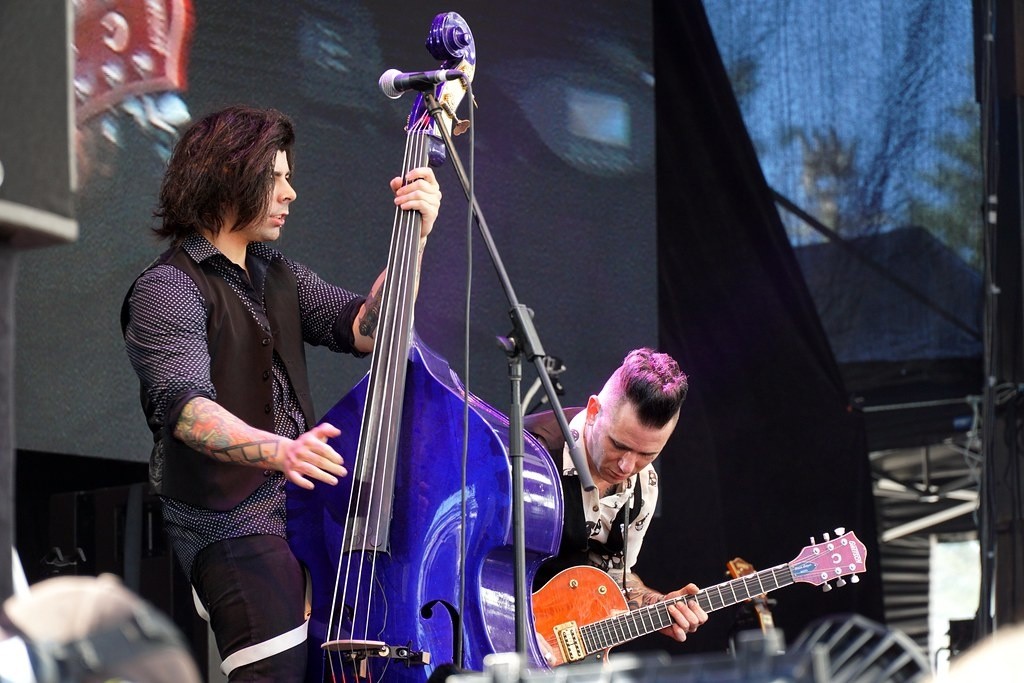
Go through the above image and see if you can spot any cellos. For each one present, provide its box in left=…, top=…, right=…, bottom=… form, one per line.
left=280, top=10, right=566, bottom=683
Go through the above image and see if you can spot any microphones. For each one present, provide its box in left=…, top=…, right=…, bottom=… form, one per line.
left=379, top=68, right=462, bottom=99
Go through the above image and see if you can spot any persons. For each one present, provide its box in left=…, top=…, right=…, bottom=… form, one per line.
left=521, top=348, right=708, bottom=646
left=120, top=104, right=441, bottom=683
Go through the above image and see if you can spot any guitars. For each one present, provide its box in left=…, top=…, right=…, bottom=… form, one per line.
left=532, top=527, right=868, bottom=668
